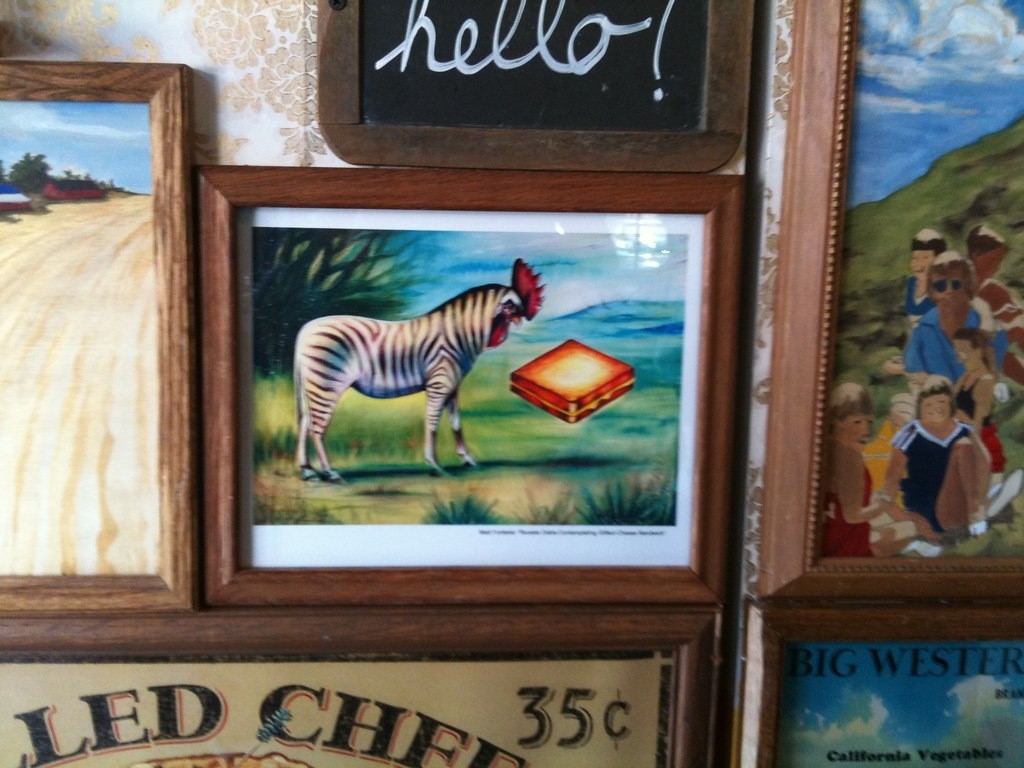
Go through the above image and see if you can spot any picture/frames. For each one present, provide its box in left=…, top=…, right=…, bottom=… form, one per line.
left=734, top=597, right=1024, bottom=768
left=0, top=62, right=198, bottom=618
left=201, top=171, right=753, bottom=604
left=0, top=610, right=722, bottom=768
left=757, top=0, right=1024, bottom=601
left=313, top=0, right=755, bottom=142
